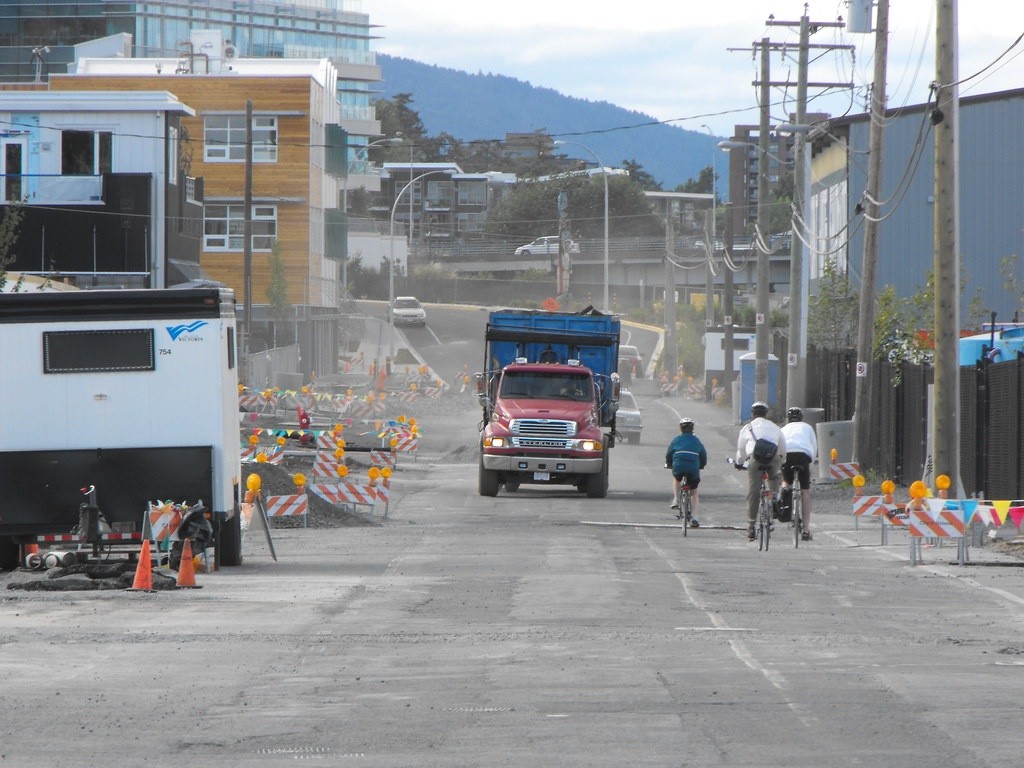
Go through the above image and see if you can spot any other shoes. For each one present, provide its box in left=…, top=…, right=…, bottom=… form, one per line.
left=771, top=495, right=779, bottom=520
left=748, top=527, right=755, bottom=538
left=670, top=498, right=680, bottom=508
left=690, top=519, right=700, bottom=528
left=801, top=530, right=812, bottom=541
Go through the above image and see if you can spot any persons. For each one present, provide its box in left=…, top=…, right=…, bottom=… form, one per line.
left=737, top=401, right=785, bottom=538
left=560, top=379, right=582, bottom=396
left=665, top=417, right=707, bottom=528
left=778, top=406, right=818, bottom=540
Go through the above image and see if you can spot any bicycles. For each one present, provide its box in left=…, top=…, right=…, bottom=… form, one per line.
left=664, top=465, right=705, bottom=538
left=734, top=463, right=775, bottom=552
left=786, top=465, right=812, bottom=549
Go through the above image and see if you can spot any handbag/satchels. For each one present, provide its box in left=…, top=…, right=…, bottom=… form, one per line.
left=753, top=438, right=778, bottom=464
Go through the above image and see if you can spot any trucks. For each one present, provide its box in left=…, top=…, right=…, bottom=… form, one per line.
left=478, top=308, right=621, bottom=497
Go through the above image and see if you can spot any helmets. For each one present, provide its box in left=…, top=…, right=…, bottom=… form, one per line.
left=751, top=400, right=769, bottom=413
left=679, top=418, right=695, bottom=430
left=787, top=406, right=803, bottom=420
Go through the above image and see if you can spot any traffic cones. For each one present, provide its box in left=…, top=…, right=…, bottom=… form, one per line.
left=126, top=539, right=156, bottom=593
left=174, top=539, right=203, bottom=590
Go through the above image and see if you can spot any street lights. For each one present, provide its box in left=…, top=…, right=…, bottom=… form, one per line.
left=777, top=122, right=885, bottom=477
left=396, top=131, right=413, bottom=244
left=718, top=139, right=804, bottom=419
left=343, top=137, right=403, bottom=301
left=554, top=140, right=611, bottom=311
left=388, top=170, right=458, bottom=304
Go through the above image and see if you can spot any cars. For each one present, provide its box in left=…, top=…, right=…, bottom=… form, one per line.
left=386, top=296, right=426, bottom=327
left=618, top=345, right=645, bottom=376
left=614, top=391, right=643, bottom=444
left=515, top=236, right=580, bottom=255
